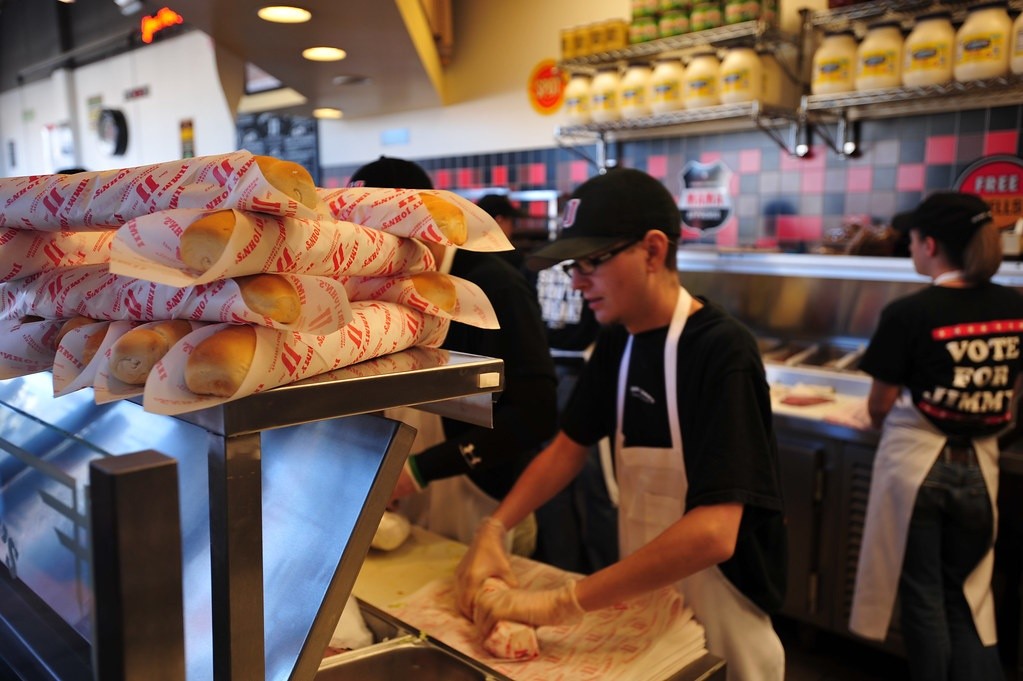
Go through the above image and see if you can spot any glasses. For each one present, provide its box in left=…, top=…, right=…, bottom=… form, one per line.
left=562, top=236, right=675, bottom=281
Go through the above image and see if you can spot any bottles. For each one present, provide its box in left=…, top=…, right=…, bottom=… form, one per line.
left=181, top=121, right=196, bottom=158
left=564, top=0, right=1022, bottom=124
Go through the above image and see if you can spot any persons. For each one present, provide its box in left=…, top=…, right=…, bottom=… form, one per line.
left=742, top=200, right=910, bottom=258
left=867, top=191, right=1023, bottom=681
left=344, top=156, right=597, bottom=570
left=454, top=165, right=790, bottom=681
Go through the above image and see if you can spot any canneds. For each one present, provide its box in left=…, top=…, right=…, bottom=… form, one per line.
left=563, top=0, right=1023, bottom=125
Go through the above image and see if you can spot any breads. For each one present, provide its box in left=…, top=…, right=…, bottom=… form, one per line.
left=55, top=153, right=468, bottom=398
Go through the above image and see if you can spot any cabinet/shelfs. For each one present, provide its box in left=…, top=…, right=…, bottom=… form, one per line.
left=796, top=0, right=1023, bottom=157
left=817, top=439, right=1023, bottom=657
left=768, top=422, right=815, bottom=625
left=554, top=0, right=818, bottom=177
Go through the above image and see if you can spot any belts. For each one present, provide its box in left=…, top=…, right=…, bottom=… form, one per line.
left=939, top=447, right=977, bottom=464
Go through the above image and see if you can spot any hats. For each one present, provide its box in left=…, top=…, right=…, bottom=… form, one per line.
left=478, top=193, right=525, bottom=218
left=528, top=168, right=681, bottom=274
left=346, top=156, right=433, bottom=190
left=891, top=192, right=994, bottom=238
